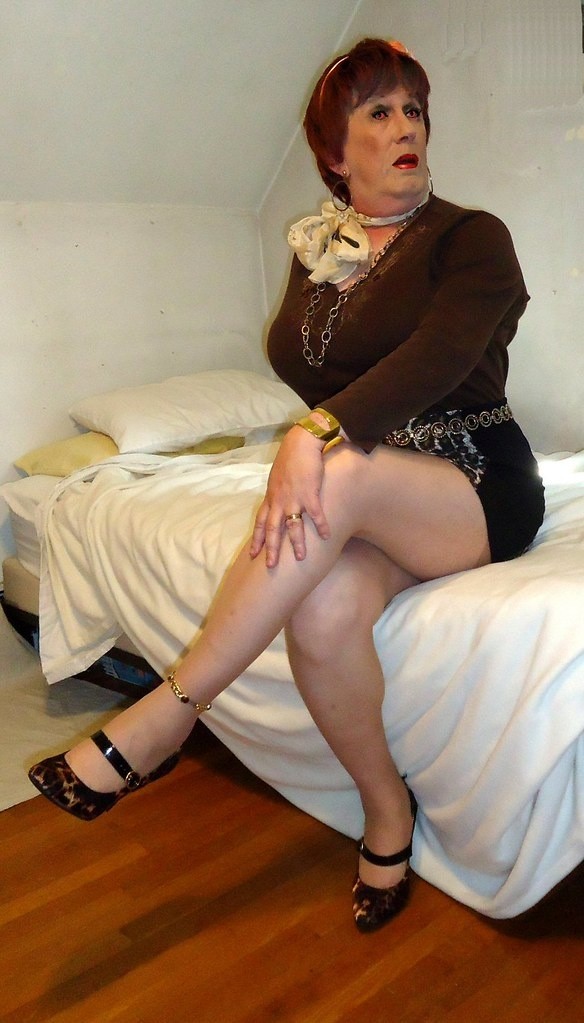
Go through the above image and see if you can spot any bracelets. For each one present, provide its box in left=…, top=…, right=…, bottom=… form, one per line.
left=293, top=408, right=346, bottom=454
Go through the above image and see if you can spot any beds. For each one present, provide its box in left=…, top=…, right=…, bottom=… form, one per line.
left=0, top=435, right=584, bottom=920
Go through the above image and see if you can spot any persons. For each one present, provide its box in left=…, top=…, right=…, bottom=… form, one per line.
left=28, top=37, right=545, bottom=934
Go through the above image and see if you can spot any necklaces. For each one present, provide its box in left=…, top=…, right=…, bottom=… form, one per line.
left=302, top=207, right=422, bottom=368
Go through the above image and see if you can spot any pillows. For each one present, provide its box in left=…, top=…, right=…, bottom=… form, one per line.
left=12, top=429, right=246, bottom=478
left=67, top=366, right=311, bottom=455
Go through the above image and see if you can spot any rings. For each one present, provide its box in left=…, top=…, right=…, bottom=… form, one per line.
left=285, top=514, right=302, bottom=521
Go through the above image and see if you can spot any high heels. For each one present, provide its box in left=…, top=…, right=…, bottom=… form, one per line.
left=349, top=774, right=419, bottom=934
left=28, top=728, right=183, bottom=822
